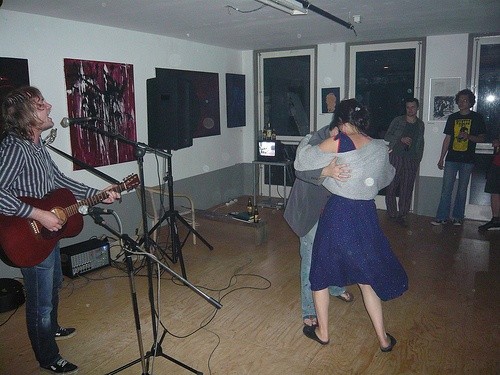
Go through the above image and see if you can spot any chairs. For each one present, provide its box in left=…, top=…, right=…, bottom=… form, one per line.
left=136, top=186, right=197, bottom=246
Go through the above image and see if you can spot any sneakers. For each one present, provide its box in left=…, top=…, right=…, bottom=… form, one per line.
left=40, top=357, right=80, bottom=375
left=55, top=326, right=77, bottom=340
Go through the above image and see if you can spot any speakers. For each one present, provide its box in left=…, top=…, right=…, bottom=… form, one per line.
left=146, top=76, right=193, bottom=151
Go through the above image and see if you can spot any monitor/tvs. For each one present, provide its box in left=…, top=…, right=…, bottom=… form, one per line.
left=257, top=140, right=282, bottom=162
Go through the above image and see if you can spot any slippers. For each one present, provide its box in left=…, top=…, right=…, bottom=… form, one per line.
left=303, top=325, right=330, bottom=345
left=303, top=315, right=319, bottom=327
left=380, top=333, right=397, bottom=352
left=335, top=292, right=354, bottom=303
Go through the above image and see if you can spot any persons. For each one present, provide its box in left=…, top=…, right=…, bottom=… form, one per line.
left=0, top=87, right=121, bottom=375
left=283, top=88, right=500, bottom=352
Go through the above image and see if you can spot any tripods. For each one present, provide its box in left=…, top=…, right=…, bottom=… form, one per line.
left=83, top=124, right=223, bottom=374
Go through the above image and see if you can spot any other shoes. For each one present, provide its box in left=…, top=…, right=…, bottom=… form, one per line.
left=478, top=217, right=500, bottom=231
left=398, top=215, right=408, bottom=225
left=453, top=218, right=462, bottom=225
left=431, top=219, right=447, bottom=226
left=388, top=212, right=397, bottom=218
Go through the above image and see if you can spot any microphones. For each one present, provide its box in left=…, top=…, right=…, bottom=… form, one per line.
left=60, top=116, right=99, bottom=128
left=78, top=205, right=115, bottom=215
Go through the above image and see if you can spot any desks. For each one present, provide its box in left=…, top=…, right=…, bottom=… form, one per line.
left=252, top=160, right=290, bottom=210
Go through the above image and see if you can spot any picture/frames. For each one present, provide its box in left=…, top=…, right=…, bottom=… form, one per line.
left=428, top=76, right=461, bottom=124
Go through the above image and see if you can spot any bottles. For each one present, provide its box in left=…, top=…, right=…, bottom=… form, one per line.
left=254, top=209, right=259, bottom=223
left=247, top=200, right=252, bottom=215
left=259, top=124, right=276, bottom=141
left=494, top=132, right=500, bottom=154
left=458, top=126, right=465, bottom=142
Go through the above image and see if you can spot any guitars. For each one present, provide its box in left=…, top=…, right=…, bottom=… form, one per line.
left=0, top=171, right=141, bottom=269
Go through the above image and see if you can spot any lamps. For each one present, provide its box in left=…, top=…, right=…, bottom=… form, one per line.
left=255, top=0, right=309, bottom=16
